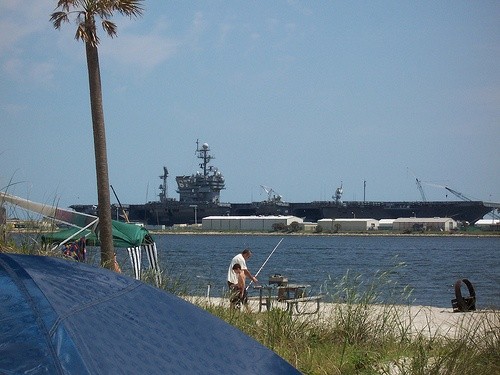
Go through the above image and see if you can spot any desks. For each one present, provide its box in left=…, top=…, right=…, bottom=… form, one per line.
left=253, top=284, right=311, bottom=314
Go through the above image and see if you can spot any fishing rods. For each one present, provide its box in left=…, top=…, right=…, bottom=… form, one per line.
left=235, top=230, right=296, bottom=306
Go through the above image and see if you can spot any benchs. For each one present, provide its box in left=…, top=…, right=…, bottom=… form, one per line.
left=247, top=296, right=279, bottom=311
left=281, top=296, right=322, bottom=315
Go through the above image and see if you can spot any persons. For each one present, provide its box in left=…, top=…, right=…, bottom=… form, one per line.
left=229, top=264, right=251, bottom=312
left=228, top=249, right=258, bottom=309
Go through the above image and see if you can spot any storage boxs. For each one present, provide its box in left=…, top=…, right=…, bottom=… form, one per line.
left=269, top=273, right=288, bottom=284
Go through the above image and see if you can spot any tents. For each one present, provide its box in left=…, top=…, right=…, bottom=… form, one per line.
left=41, top=218, right=154, bottom=247
